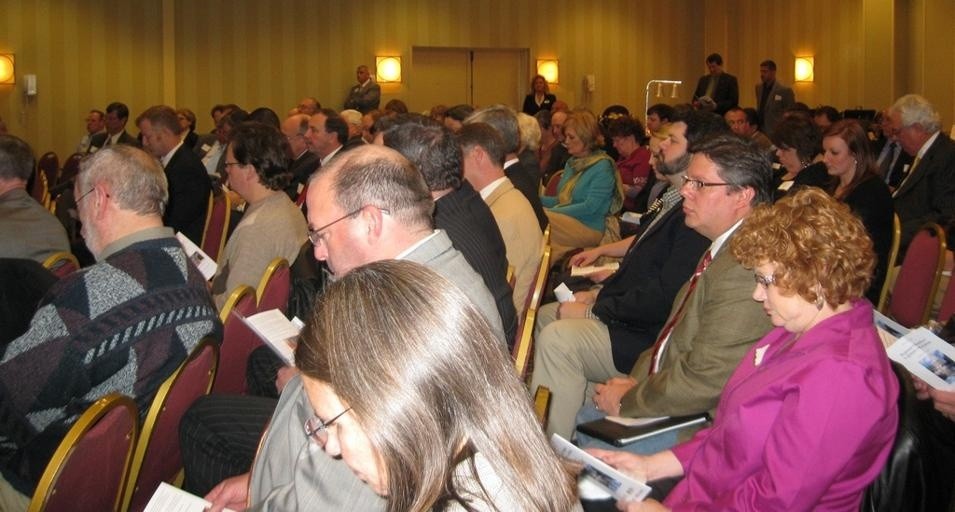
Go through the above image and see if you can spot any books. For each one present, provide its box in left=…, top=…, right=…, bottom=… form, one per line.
left=575, top=415, right=710, bottom=447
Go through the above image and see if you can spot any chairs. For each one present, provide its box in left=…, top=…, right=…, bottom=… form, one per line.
left=200, top=190, right=213, bottom=251
left=49, top=200, right=56, bottom=214
left=211, top=283, right=257, bottom=395
left=886, top=222, right=945, bottom=328
left=29, top=168, right=48, bottom=204
left=203, top=194, right=230, bottom=264
left=525, top=244, right=551, bottom=310
left=27, top=390, right=139, bottom=512
left=511, top=307, right=535, bottom=381
left=542, top=223, right=553, bottom=247
left=864, top=210, right=900, bottom=312
left=44, top=193, right=51, bottom=210
left=252, top=257, right=296, bottom=350
left=61, top=152, right=85, bottom=183
left=929, top=249, right=955, bottom=331
left=545, top=171, right=564, bottom=196
left=121, top=335, right=221, bottom=512
left=38, top=152, right=59, bottom=189
left=533, top=385, right=552, bottom=432
left=41, top=252, right=79, bottom=279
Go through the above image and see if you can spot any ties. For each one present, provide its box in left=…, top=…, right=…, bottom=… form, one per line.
left=294, top=164, right=324, bottom=211
left=875, top=139, right=897, bottom=183
left=648, top=250, right=712, bottom=378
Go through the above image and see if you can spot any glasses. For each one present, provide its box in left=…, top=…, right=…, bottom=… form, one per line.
left=83, top=116, right=98, bottom=123
left=69, top=186, right=113, bottom=204
left=223, top=161, right=249, bottom=169
left=306, top=204, right=390, bottom=247
left=304, top=405, right=355, bottom=437
left=682, top=172, right=761, bottom=195
left=285, top=135, right=304, bottom=142
left=890, top=125, right=903, bottom=138
left=753, top=272, right=781, bottom=287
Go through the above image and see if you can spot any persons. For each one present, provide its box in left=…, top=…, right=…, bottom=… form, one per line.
left=134, top=105, right=214, bottom=251
left=754, top=55, right=795, bottom=128
left=203, top=145, right=510, bottom=512
left=343, top=62, right=379, bottom=112
left=723, top=107, right=781, bottom=161
left=870, top=94, right=952, bottom=270
left=462, top=103, right=547, bottom=232
left=538, top=108, right=618, bottom=273
left=448, top=124, right=545, bottom=336
left=514, top=113, right=543, bottom=192
left=523, top=75, right=555, bottom=117
left=820, top=116, right=897, bottom=312
left=88, top=103, right=139, bottom=146
left=599, top=103, right=631, bottom=124
left=810, top=105, right=842, bottom=133
left=868, top=111, right=915, bottom=194
left=542, top=113, right=573, bottom=185
left=206, top=95, right=321, bottom=134
left=294, top=260, right=583, bottom=512
left=194, top=125, right=310, bottom=319
left=569, top=136, right=781, bottom=502
left=305, top=109, right=356, bottom=178
left=0, top=143, right=224, bottom=512
left=337, top=111, right=371, bottom=146
left=176, top=109, right=196, bottom=133
left=527, top=108, right=729, bottom=462
left=689, top=48, right=739, bottom=115
left=769, top=112, right=840, bottom=203
left=579, top=183, right=899, bottom=512
left=908, top=305, right=955, bottom=509
left=645, top=102, right=672, bottom=136
left=604, top=122, right=656, bottom=213
left=791, top=101, right=811, bottom=115
left=370, top=122, right=519, bottom=357
left=76, top=111, right=106, bottom=154
left=276, top=112, right=315, bottom=187
left=363, top=95, right=472, bottom=133
left=0, top=132, right=72, bottom=286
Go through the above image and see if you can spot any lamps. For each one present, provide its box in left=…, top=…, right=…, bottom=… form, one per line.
left=374, top=56, right=402, bottom=83
left=0, top=53, right=16, bottom=88
left=792, top=56, right=816, bottom=86
left=535, top=56, right=559, bottom=86
